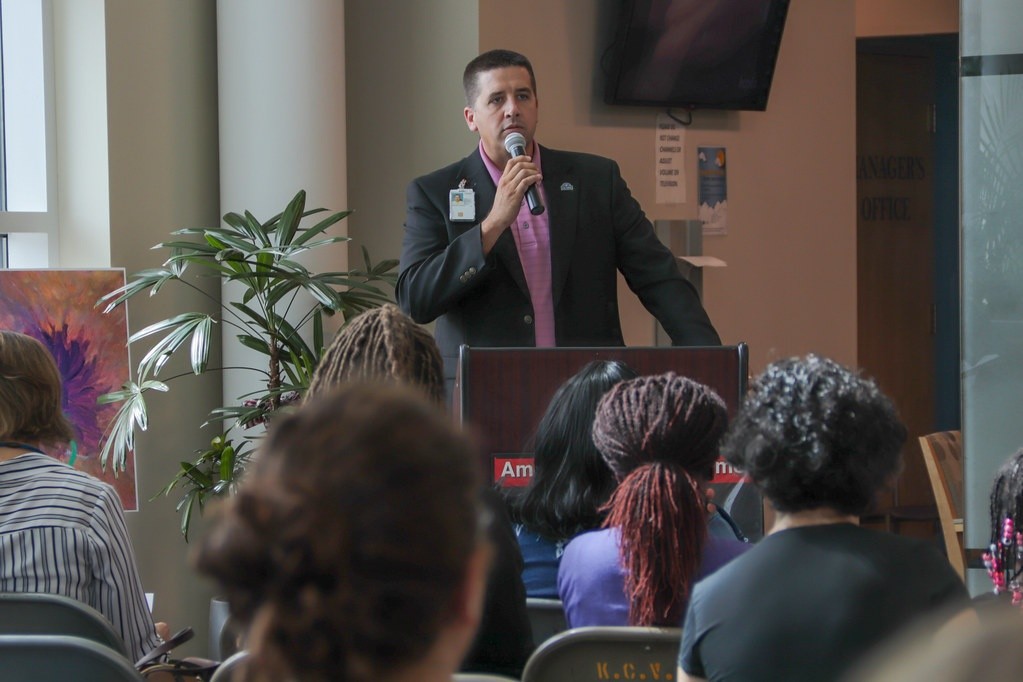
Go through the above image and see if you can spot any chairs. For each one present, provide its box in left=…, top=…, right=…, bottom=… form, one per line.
left=447, top=595, right=682, bottom=682
left=0, top=592, right=247, bottom=682
left=918, top=430, right=967, bottom=585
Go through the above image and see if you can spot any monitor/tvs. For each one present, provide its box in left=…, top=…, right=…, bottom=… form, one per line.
left=605, top=0, right=790, bottom=112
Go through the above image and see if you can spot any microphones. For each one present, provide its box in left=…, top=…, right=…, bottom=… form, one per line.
left=505, top=133, right=545, bottom=216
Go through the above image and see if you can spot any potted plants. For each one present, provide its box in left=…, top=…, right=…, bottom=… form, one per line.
left=92, top=189, right=399, bottom=658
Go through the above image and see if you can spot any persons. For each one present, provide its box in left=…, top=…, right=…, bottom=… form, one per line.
left=519, top=359, right=639, bottom=600
left=556, top=372, right=753, bottom=629
left=0, top=330, right=168, bottom=672
left=304, top=306, right=533, bottom=682
left=193, top=379, right=492, bottom=681
left=676, top=351, right=968, bottom=682
left=451, top=194, right=463, bottom=205
left=395, top=48, right=722, bottom=409
left=935, top=448, right=1022, bottom=647
